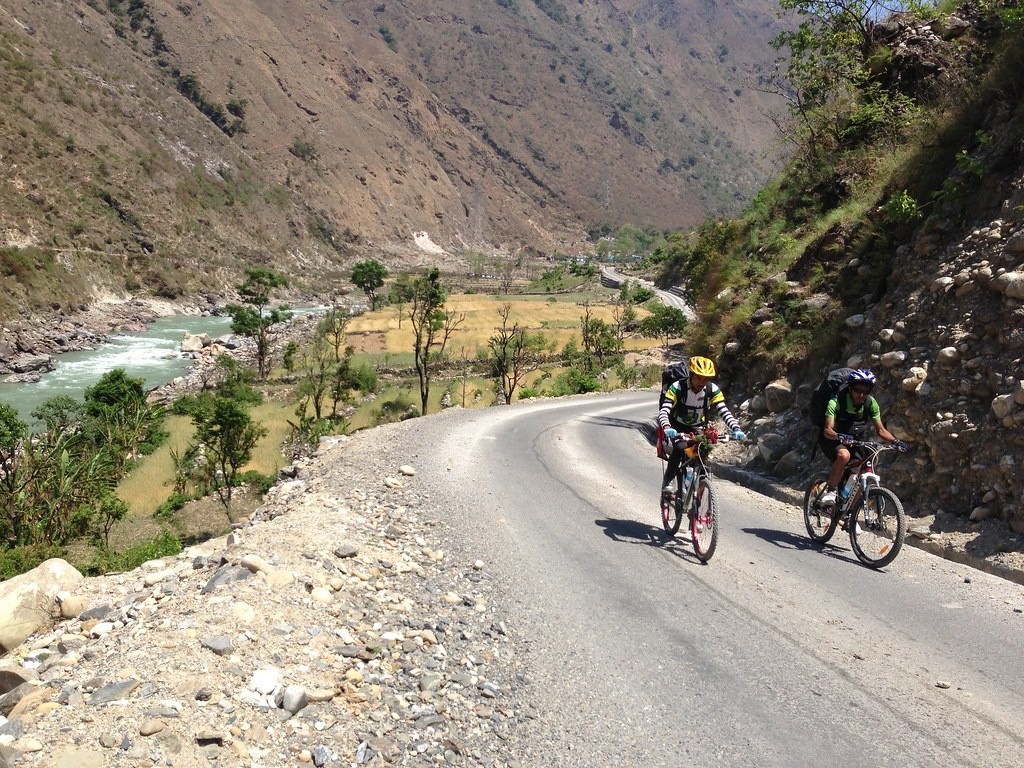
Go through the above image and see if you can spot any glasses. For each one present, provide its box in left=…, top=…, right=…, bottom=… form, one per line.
left=851, top=386, right=870, bottom=394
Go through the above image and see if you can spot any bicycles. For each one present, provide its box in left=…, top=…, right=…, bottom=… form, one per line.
left=660, top=432, right=753, bottom=563
left=802, top=437, right=906, bottom=568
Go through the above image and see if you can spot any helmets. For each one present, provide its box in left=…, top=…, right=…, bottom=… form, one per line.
left=689, top=356, right=716, bottom=376
left=849, top=369, right=877, bottom=385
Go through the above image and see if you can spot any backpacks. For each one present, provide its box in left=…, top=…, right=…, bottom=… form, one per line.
left=810, top=367, right=872, bottom=428
left=659, top=362, right=712, bottom=426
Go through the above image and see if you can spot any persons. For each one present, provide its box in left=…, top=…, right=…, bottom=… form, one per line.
left=657, top=355, right=750, bottom=532
left=817, top=369, right=909, bottom=538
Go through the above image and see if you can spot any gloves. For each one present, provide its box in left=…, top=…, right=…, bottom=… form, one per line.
left=837, top=433, right=853, bottom=447
left=892, top=439, right=908, bottom=453
left=663, top=428, right=677, bottom=438
left=731, top=430, right=747, bottom=445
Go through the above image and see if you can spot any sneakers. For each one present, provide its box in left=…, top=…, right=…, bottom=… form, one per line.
left=822, top=490, right=837, bottom=504
left=662, top=475, right=673, bottom=493
left=855, top=523, right=862, bottom=536
left=689, top=519, right=703, bottom=531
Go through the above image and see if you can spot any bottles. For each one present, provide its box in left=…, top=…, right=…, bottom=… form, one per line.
left=841, top=473, right=859, bottom=498
left=685, top=467, right=694, bottom=487
